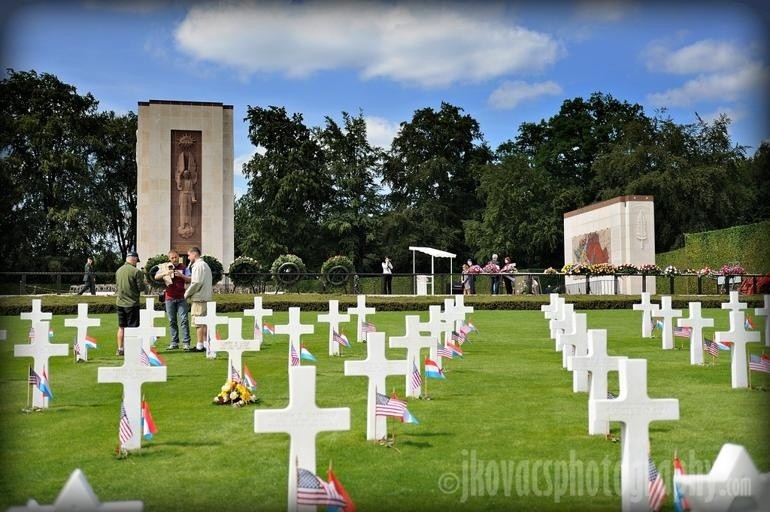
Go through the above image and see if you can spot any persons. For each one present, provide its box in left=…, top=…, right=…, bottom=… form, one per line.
left=115, top=251, right=147, bottom=354
left=501, top=257, right=518, bottom=294
left=463, top=258, right=477, bottom=295
left=153, top=248, right=192, bottom=350
left=488, top=253, right=501, bottom=294
left=182, top=246, right=213, bottom=352
left=175, top=168, right=198, bottom=230
left=381, top=256, right=394, bottom=295
left=531, top=276, right=540, bottom=294
left=461, top=263, right=471, bottom=294
left=77, top=257, right=97, bottom=295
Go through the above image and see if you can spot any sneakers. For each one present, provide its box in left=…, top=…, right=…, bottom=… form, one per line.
left=116, top=349, right=124, bottom=355
left=166, top=345, right=206, bottom=352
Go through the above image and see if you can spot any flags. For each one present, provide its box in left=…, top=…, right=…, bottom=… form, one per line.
left=26, top=326, right=164, bottom=400
left=647, top=311, right=769, bottom=374
left=647, top=449, right=668, bottom=511
left=206, top=320, right=275, bottom=393
left=289, top=320, right=377, bottom=366
left=140, top=397, right=159, bottom=443
left=119, top=396, right=134, bottom=446
left=391, top=393, right=420, bottom=425
left=296, top=467, right=348, bottom=508
left=327, top=465, right=358, bottom=512
left=411, top=322, right=479, bottom=389
left=673, top=454, right=689, bottom=511
left=375, top=391, right=407, bottom=418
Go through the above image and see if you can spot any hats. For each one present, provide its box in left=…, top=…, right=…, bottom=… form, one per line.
left=127, top=252, right=140, bottom=262
